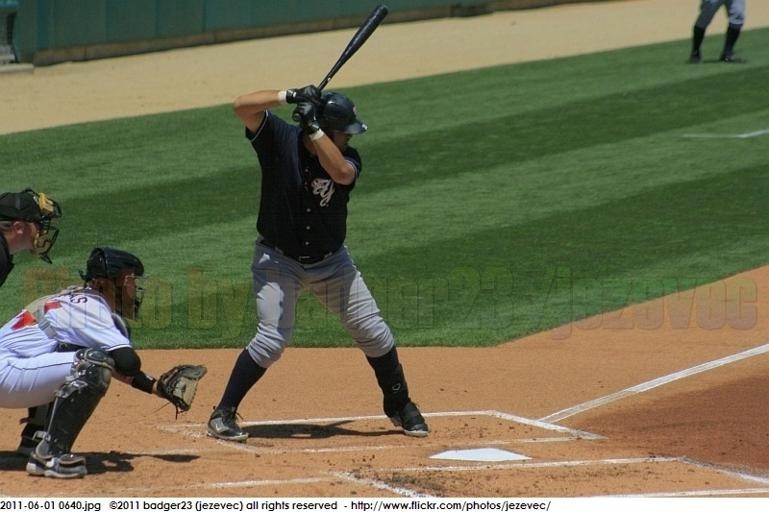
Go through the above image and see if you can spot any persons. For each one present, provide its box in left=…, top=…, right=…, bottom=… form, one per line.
left=686, top=0, right=749, bottom=64
left=208, top=85, right=430, bottom=440
left=0, top=247, right=208, bottom=480
left=0, top=187, right=63, bottom=286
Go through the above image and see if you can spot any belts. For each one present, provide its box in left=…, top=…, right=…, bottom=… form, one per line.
left=255, top=231, right=334, bottom=266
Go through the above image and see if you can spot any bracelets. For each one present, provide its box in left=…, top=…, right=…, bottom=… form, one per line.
left=278, top=90, right=286, bottom=105
left=309, top=128, right=325, bottom=142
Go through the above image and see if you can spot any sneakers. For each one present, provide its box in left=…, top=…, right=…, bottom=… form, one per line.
left=206, top=406, right=250, bottom=440
left=383, top=397, right=430, bottom=437
left=16, top=436, right=88, bottom=479
left=721, top=52, right=741, bottom=63
left=690, top=52, right=701, bottom=64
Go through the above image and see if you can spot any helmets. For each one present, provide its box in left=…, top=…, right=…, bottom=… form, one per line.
left=1, top=187, right=61, bottom=264
left=317, top=92, right=368, bottom=136
left=78, top=247, right=144, bottom=322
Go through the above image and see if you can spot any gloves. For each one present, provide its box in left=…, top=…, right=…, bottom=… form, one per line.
left=285, top=85, right=327, bottom=137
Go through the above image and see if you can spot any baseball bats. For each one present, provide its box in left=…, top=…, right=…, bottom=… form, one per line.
left=293, top=3, right=389, bottom=122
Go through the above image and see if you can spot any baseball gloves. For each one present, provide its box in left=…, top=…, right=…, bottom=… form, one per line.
left=157, top=365, right=207, bottom=421
